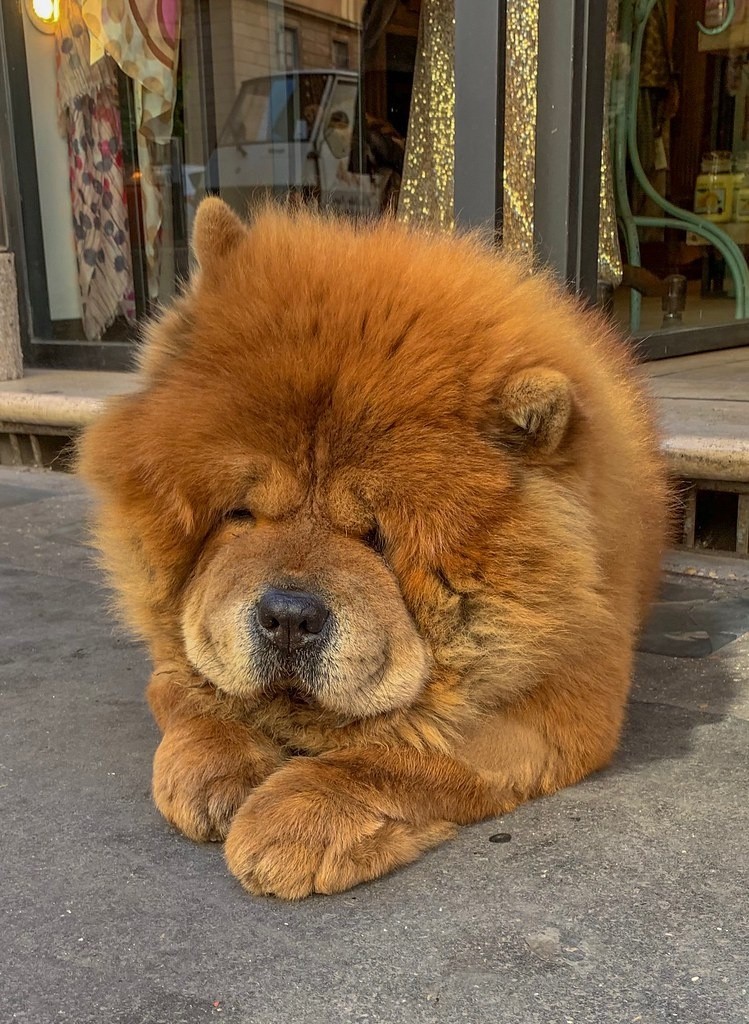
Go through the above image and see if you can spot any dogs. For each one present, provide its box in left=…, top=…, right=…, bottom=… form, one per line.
left=67, top=192, right=671, bottom=901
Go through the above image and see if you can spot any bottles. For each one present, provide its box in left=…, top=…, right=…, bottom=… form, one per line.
left=734, top=151, right=749, bottom=223
left=694, top=152, right=734, bottom=223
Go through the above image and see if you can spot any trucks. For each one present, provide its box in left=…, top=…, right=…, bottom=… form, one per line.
left=205, top=70, right=401, bottom=227
